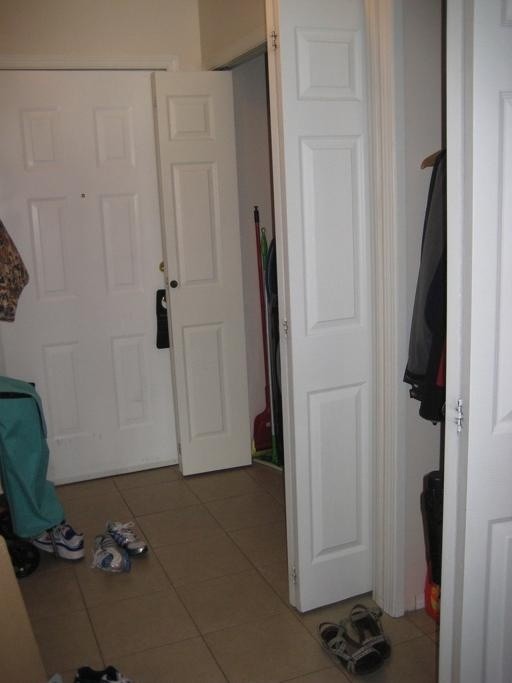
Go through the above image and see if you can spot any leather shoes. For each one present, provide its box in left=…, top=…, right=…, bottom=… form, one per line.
left=8, top=537, right=39, bottom=578
left=0, top=494, right=13, bottom=536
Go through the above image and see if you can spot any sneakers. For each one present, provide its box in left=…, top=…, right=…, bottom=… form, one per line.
left=105, top=520, right=148, bottom=554
left=74, top=664, right=134, bottom=683
left=90, top=533, right=131, bottom=572
left=28, top=523, right=85, bottom=559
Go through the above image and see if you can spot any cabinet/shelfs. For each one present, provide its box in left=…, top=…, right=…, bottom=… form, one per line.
left=272, top=0, right=511, bottom=683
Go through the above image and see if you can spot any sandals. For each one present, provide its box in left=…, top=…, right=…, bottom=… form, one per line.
left=349, top=604, right=391, bottom=658
left=317, top=621, right=384, bottom=677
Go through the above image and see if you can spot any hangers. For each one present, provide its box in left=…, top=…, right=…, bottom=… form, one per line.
left=420, top=149, right=442, bottom=169
